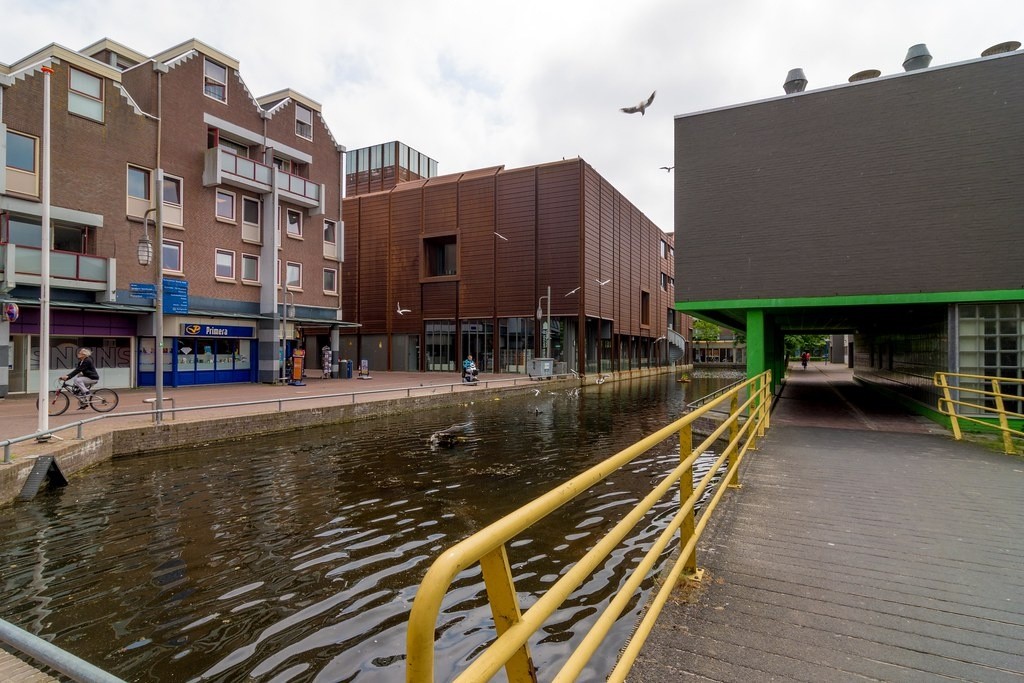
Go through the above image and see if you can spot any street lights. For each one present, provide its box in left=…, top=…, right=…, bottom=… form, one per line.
left=282, top=280, right=296, bottom=386
left=824, top=339, right=830, bottom=365
left=536, top=286, right=550, bottom=357
left=136, top=169, right=166, bottom=422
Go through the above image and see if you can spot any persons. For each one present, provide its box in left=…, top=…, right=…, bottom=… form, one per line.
left=462, top=355, right=479, bottom=381
left=61, top=349, right=99, bottom=410
left=802, top=352, right=810, bottom=367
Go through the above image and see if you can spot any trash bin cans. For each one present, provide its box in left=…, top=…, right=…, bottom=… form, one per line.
left=347, top=360, right=353, bottom=378
left=340, top=360, right=348, bottom=378
left=528, top=358, right=553, bottom=380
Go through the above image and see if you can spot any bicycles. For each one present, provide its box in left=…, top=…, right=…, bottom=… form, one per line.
left=802, top=356, right=809, bottom=371
left=36, top=375, right=119, bottom=416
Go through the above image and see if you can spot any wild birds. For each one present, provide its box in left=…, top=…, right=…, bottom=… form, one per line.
left=396, top=301, right=412, bottom=315
left=565, top=286, right=580, bottom=296
left=660, top=166, right=674, bottom=172
left=594, top=278, right=611, bottom=286
left=620, top=90, right=656, bottom=116
left=493, top=231, right=508, bottom=241
